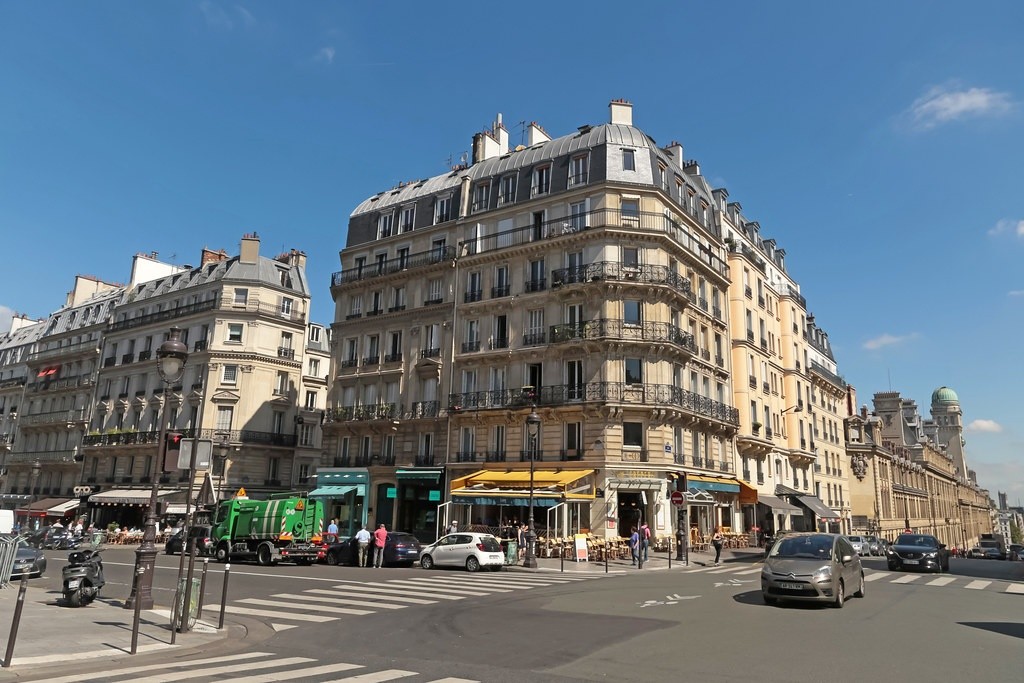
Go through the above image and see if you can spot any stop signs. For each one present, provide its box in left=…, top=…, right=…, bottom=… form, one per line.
left=905, top=530, right=911, bottom=534
left=671, top=491, right=683, bottom=505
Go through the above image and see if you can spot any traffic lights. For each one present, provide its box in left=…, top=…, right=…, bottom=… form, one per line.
left=164, top=432, right=187, bottom=474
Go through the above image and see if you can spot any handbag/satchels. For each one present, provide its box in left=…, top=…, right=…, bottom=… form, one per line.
left=713, top=533, right=723, bottom=546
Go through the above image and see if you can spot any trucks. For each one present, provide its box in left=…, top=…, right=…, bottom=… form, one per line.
left=978, top=533, right=1007, bottom=560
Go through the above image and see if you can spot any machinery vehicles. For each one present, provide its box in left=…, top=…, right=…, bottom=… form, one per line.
left=210, top=494, right=326, bottom=567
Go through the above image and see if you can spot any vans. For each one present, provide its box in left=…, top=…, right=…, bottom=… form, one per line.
left=971, top=548, right=980, bottom=559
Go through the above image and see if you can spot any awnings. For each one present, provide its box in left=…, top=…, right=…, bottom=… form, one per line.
left=14, top=497, right=80, bottom=517
left=796, top=495, right=841, bottom=523
left=88, top=489, right=185, bottom=507
left=756, top=494, right=804, bottom=516
left=308, top=485, right=357, bottom=499
left=449, top=468, right=596, bottom=506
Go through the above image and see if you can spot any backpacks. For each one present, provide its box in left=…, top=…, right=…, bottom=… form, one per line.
left=639, top=527, right=647, bottom=539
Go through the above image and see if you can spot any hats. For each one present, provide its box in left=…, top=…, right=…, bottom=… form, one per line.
left=452, top=520, right=458, bottom=524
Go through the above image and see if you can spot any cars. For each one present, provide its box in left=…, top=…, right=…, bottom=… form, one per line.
left=878, top=538, right=889, bottom=553
left=163, top=524, right=214, bottom=556
left=24, top=526, right=76, bottom=550
left=764, top=538, right=776, bottom=557
left=0, top=533, right=47, bottom=579
left=1007, top=544, right=1024, bottom=561
left=418, top=532, right=506, bottom=572
left=760, top=532, right=866, bottom=607
left=845, top=535, right=871, bottom=557
left=326, top=532, right=422, bottom=566
left=864, top=535, right=885, bottom=557
left=886, top=534, right=949, bottom=573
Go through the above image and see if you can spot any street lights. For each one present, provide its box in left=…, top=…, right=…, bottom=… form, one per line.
left=125, top=324, right=189, bottom=610
left=214, top=435, right=230, bottom=506
left=522, top=404, right=542, bottom=568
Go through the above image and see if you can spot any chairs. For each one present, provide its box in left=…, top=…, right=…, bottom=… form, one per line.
left=107, top=532, right=170, bottom=546
left=539, top=530, right=750, bottom=561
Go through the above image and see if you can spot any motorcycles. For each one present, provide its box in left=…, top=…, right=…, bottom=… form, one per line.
left=61, top=535, right=108, bottom=609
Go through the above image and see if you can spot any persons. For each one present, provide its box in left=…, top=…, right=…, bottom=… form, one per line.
left=712, top=526, right=724, bottom=564
left=372, top=524, right=387, bottom=568
left=48, top=519, right=64, bottom=528
left=328, top=518, right=343, bottom=542
left=952, top=547, right=968, bottom=559
left=511, top=522, right=528, bottom=561
left=446, top=520, right=458, bottom=544
left=355, top=525, right=371, bottom=568
left=14, top=521, right=21, bottom=530
left=630, top=526, right=644, bottom=566
left=68, top=519, right=84, bottom=537
left=642, top=521, right=651, bottom=563
